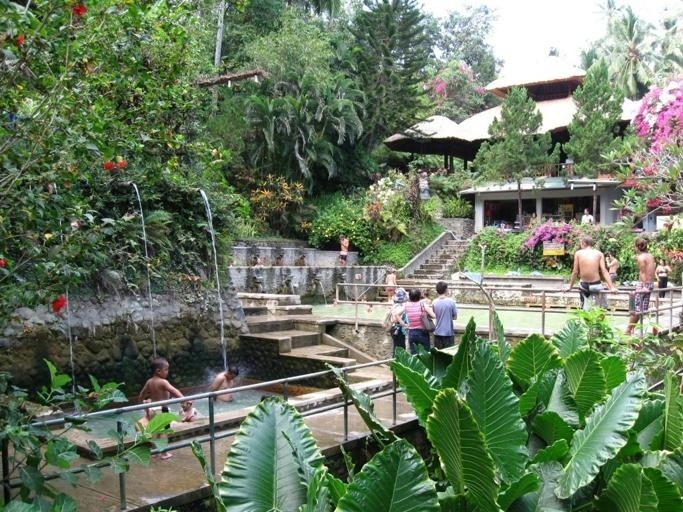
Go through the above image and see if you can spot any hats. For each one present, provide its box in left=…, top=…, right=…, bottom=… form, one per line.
left=392, top=289, right=409, bottom=302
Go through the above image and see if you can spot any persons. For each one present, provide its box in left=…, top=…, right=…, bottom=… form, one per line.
left=177, top=400, right=203, bottom=423
left=563, top=235, right=620, bottom=316
left=580, top=208, right=594, bottom=225
left=394, top=288, right=436, bottom=356
left=135, top=356, right=185, bottom=406
left=338, top=234, right=349, bottom=266
left=420, top=293, right=433, bottom=304
left=605, top=253, right=618, bottom=286
left=384, top=267, right=397, bottom=303
left=655, top=258, right=673, bottom=298
left=430, top=280, right=458, bottom=350
left=133, top=398, right=173, bottom=435
left=207, top=365, right=239, bottom=402
left=620, top=236, right=659, bottom=336
left=528, top=211, right=537, bottom=229
left=389, top=287, right=408, bottom=372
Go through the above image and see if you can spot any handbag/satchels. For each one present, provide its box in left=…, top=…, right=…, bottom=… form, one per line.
left=422, top=313, right=435, bottom=332
left=382, top=311, right=392, bottom=332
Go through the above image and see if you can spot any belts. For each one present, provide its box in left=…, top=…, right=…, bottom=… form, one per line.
left=580, top=280, right=601, bottom=285
